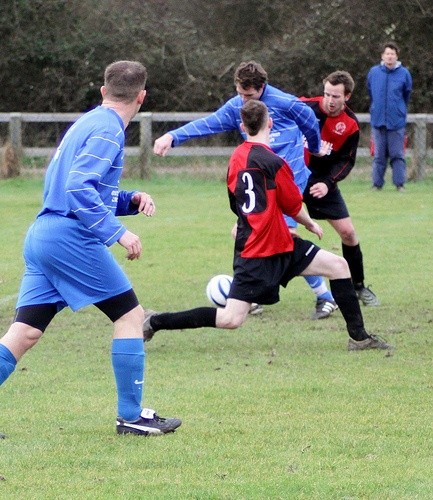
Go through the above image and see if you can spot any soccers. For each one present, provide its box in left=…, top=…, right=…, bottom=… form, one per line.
left=204, top=273, right=234, bottom=308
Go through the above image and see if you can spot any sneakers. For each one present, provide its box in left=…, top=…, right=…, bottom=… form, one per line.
left=347, top=334, right=394, bottom=350
left=312, top=299, right=338, bottom=319
left=143, top=309, right=158, bottom=342
left=116, top=408, right=182, bottom=436
left=355, top=286, right=380, bottom=306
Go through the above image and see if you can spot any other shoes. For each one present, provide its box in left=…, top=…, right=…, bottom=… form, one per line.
left=398, top=184, right=407, bottom=192
left=369, top=185, right=378, bottom=192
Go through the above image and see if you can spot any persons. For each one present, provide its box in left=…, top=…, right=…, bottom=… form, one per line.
left=294, top=71, right=381, bottom=307
left=153, top=62, right=339, bottom=320
left=0, top=60, right=182, bottom=436
left=365, top=43, right=412, bottom=192
left=141, top=100, right=391, bottom=351
